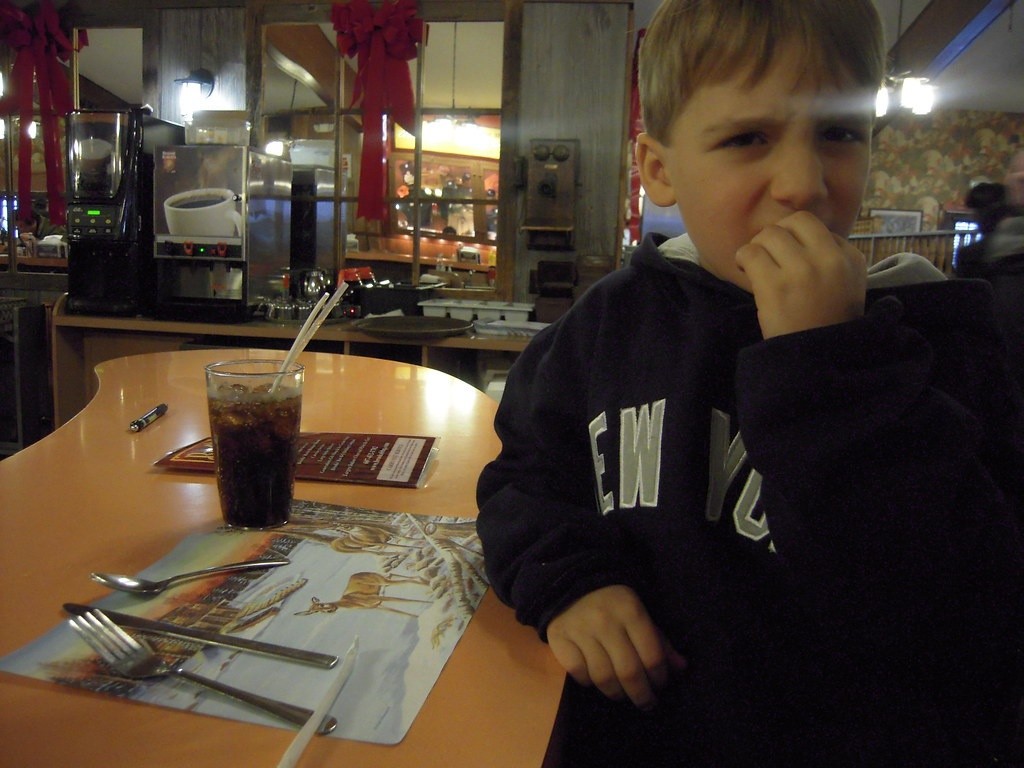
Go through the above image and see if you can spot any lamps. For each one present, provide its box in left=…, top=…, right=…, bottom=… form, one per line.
left=875, top=0, right=939, bottom=118
left=173, top=69, right=214, bottom=104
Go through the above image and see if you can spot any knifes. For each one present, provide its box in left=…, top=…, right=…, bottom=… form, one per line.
left=63, top=600, right=340, bottom=671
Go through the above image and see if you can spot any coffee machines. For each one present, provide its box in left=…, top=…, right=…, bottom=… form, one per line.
left=64, top=106, right=186, bottom=313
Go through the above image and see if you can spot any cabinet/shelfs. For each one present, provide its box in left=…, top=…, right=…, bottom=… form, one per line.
left=50, top=290, right=541, bottom=429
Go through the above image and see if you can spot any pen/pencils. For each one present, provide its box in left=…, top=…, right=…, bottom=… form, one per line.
left=130, top=404, right=168, bottom=433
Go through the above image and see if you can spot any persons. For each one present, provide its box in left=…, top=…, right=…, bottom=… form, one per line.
left=0, top=210, right=68, bottom=252
left=956, top=184, right=1024, bottom=337
left=476, top=1, right=1024, bottom=768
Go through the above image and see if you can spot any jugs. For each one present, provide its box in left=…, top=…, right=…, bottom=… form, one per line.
left=293, top=268, right=336, bottom=301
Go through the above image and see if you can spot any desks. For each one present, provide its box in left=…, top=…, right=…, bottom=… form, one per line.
left=0, top=349, right=572, bottom=766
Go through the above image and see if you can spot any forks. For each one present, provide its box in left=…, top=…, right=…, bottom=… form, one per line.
left=66, top=608, right=339, bottom=736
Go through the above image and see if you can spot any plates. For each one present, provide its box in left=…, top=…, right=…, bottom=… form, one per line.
left=352, top=314, right=474, bottom=341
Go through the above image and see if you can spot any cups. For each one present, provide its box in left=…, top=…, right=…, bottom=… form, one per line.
left=163, top=187, right=243, bottom=239
left=202, top=358, right=306, bottom=534
left=266, top=301, right=344, bottom=319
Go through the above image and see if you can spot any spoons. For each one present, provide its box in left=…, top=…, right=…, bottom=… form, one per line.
left=87, top=560, right=291, bottom=596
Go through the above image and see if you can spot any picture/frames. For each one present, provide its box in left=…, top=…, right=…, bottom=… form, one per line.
left=869, top=208, right=923, bottom=233
